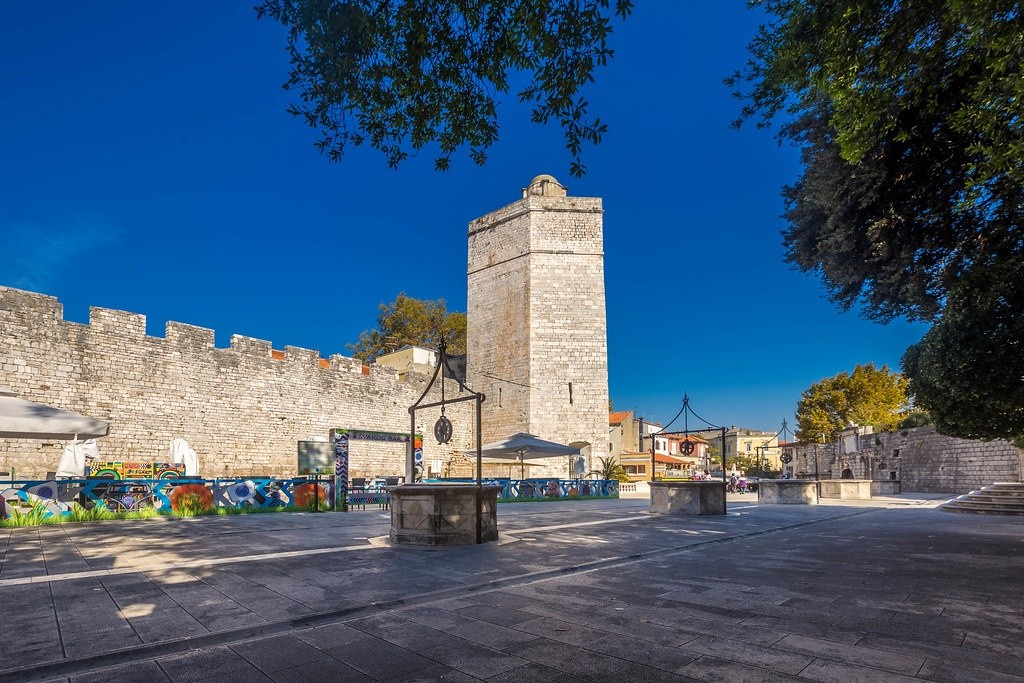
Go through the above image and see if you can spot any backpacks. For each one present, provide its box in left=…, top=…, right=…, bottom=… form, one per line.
left=730, top=476, right=736, bottom=485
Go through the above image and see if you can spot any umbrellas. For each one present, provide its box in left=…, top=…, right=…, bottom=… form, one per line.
left=463, top=432, right=580, bottom=480
left=0, top=388, right=110, bottom=440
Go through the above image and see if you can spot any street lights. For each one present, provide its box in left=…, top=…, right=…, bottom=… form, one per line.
left=704, top=445, right=709, bottom=474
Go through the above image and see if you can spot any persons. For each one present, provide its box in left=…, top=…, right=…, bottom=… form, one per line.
left=729, top=473, right=736, bottom=493
left=701, top=469, right=712, bottom=480
left=738, top=474, right=747, bottom=494
left=693, top=471, right=701, bottom=480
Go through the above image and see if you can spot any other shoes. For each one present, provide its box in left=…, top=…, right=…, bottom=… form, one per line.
left=740, top=492, right=741, bottom=494
left=742, top=492, right=744, bottom=494
